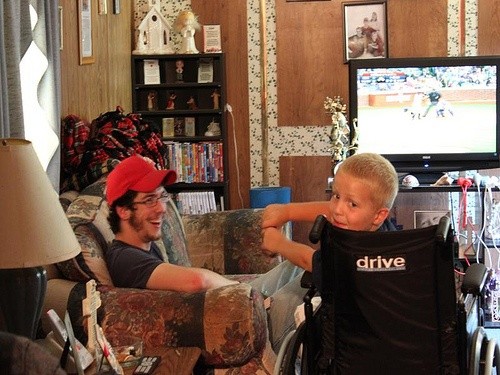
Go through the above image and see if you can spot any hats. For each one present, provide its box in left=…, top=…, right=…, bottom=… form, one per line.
left=105, top=153, right=177, bottom=210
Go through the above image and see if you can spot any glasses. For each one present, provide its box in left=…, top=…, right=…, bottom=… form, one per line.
left=123, top=192, right=173, bottom=208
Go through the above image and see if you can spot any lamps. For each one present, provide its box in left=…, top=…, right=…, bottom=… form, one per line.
left=0, top=138, right=82, bottom=337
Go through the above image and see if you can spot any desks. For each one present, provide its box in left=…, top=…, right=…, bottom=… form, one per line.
left=84, top=346, right=201, bottom=375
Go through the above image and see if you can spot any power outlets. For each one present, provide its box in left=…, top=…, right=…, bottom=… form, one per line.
left=450, top=192, right=475, bottom=244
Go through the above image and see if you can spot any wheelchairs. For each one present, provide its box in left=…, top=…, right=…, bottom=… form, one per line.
left=272, top=211, right=500, bottom=375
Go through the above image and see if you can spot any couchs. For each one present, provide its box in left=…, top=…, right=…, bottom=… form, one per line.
left=42, top=179, right=285, bottom=375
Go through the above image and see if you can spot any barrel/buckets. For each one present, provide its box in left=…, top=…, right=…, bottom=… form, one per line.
left=249, top=186, right=291, bottom=209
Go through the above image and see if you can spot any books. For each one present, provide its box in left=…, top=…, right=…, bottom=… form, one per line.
left=177, top=190, right=216, bottom=215
left=165, top=142, right=224, bottom=183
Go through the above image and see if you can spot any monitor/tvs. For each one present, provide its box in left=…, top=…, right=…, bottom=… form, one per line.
left=348, top=54, right=499, bottom=184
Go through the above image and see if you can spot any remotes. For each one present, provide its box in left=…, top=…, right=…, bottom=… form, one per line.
left=133, top=355, right=161, bottom=375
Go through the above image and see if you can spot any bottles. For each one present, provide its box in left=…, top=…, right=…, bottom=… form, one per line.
left=483, top=269, right=499, bottom=322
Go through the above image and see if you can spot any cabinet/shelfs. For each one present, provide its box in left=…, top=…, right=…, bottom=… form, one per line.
left=326, top=184, right=500, bottom=269
left=131, top=52, right=230, bottom=214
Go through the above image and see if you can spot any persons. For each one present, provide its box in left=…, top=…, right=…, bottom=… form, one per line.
left=261, top=152, right=399, bottom=272
left=187, top=96, right=196, bottom=110
left=348, top=12, right=384, bottom=58
left=166, top=94, right=176, bottom=109
left=211, top=89, right=221, bottom=109
left=175, top=60, right=184, bottom=79
left=148, top=93, right=154, bottom=110
left=105, top=155, right=309, bottom=354
left=411, top=89, right=454, bottom=120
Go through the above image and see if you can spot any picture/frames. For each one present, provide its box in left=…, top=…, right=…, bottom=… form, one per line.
left=342, top=0, right=388, bottom=64
left=414, top=210, right=450, bottom=229
left=58, top=0, right=121, bottom=65
